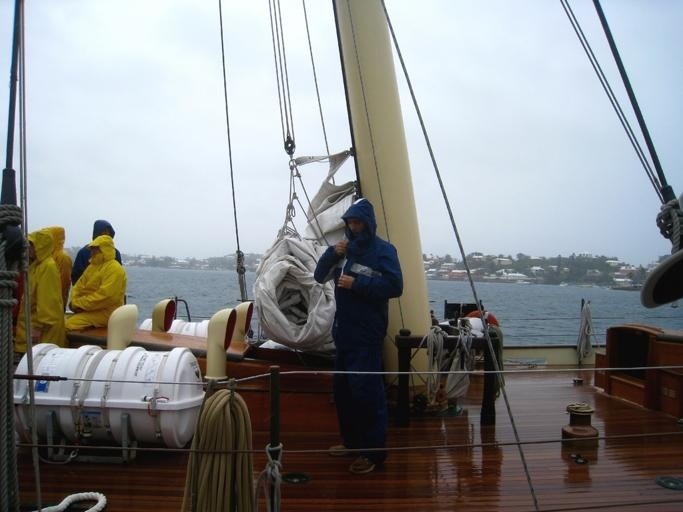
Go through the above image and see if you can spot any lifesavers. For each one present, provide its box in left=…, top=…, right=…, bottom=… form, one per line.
left=467, top=310, right=498, bottom=325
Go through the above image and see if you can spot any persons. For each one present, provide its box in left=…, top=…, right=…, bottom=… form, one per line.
left=42, top=226, right=73, bottom=313
left=314, top=197, right=403, bottom=474
left=14, top=229, right=66, bottom=354
left=71, top=220, right=122, bottom=286
left=61, top=235, right=128, bottom=331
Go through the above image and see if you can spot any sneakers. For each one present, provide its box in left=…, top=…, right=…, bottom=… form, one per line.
left=347, top=452, right=380, bottom=475
left=327, top=441, right=354, bottom=457
